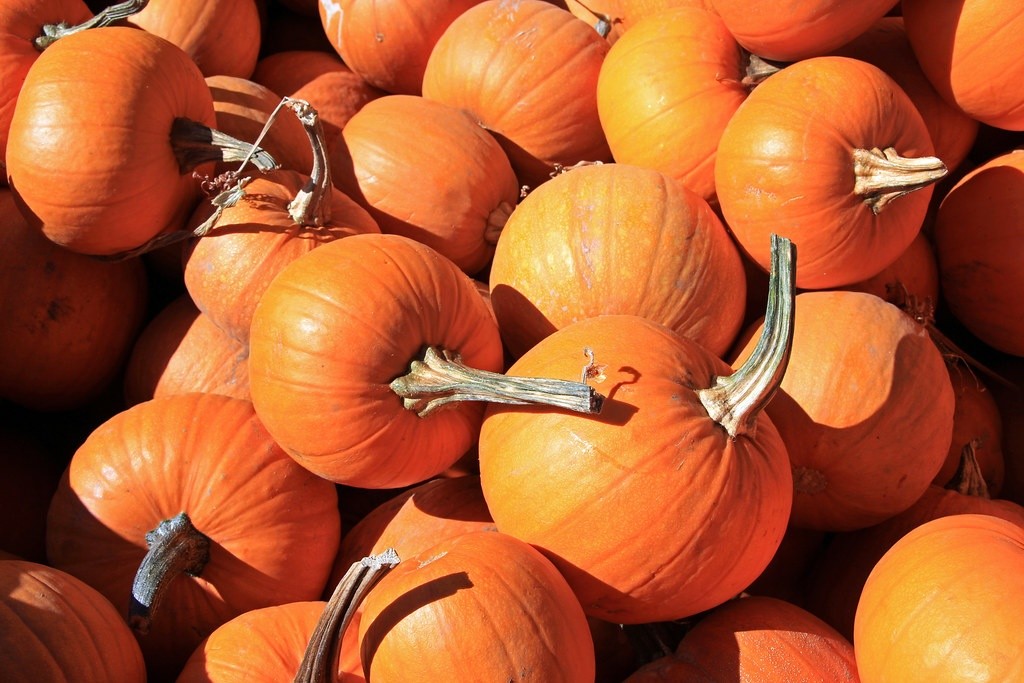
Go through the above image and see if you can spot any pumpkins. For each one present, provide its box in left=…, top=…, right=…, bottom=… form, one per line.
left=0, top=0, right=1024, bottom=683
